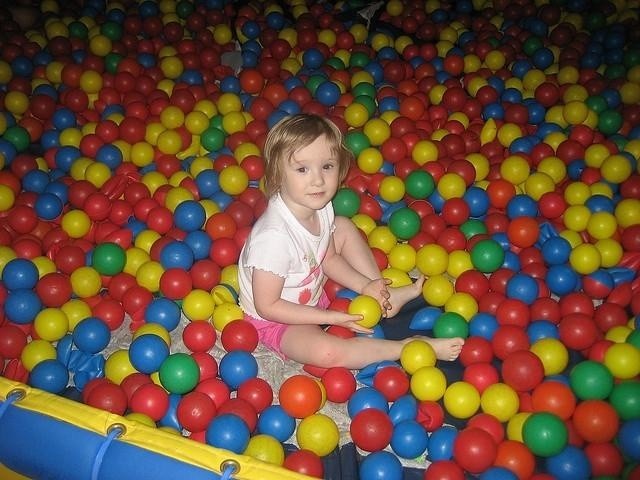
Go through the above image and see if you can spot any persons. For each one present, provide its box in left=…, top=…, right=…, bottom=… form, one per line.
left=238, top=114, right=464, bottom=369
